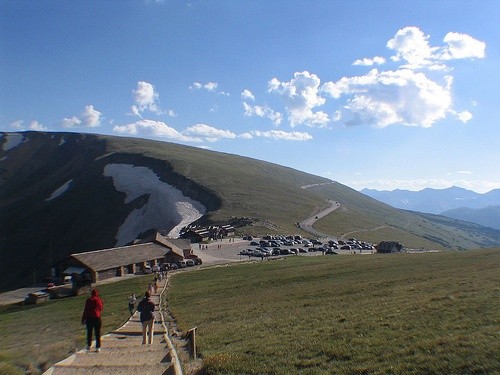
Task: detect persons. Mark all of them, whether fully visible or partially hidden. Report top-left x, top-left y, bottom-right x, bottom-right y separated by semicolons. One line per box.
217;244;219;249
229;239;231;242
266;252;269;258
147;270;169;297
128;293;136;316
82;289;103;353
233;239;234;242
261;254;264;261
220;245;221;249
206;245;207;249
202;245;204;249
137;291;156;345
199;244;201;249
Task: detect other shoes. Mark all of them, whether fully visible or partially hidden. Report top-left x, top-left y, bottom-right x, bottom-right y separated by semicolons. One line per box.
95;348;101;353
141;337;153;345
86;345;92;350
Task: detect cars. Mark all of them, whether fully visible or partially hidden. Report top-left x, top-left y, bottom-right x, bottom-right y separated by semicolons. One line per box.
240;235;373;255
